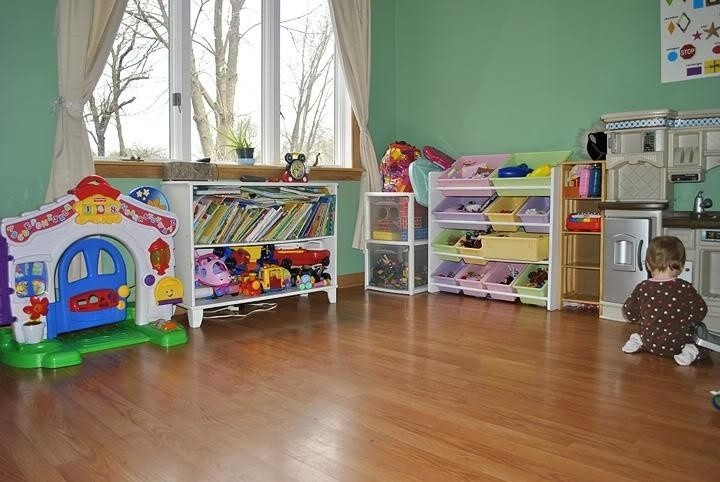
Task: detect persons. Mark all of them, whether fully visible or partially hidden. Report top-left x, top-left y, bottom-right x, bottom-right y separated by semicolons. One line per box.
621;235;714;367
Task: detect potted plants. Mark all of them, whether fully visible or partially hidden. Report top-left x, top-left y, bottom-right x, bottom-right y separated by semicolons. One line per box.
207;119;255;159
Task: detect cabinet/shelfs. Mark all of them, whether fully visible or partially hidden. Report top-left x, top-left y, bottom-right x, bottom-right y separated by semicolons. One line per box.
557;160;605;318
423;154;562;313
164;177;338;329
364;192;427;297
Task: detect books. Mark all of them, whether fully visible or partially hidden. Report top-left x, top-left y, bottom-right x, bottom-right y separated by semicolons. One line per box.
192;185;337;245
